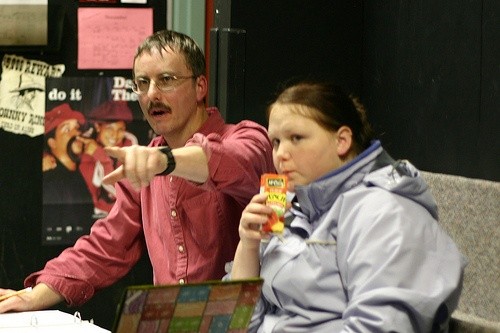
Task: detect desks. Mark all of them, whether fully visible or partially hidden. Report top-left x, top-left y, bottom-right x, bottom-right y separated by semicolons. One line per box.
0;308;110;333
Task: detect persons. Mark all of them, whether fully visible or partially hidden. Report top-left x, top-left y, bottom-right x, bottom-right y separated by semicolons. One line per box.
1;30;277;314
87;101;136;218
43;103;94;225
220;84;469;333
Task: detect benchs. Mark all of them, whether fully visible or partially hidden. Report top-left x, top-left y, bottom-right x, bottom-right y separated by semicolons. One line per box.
419;170;500;333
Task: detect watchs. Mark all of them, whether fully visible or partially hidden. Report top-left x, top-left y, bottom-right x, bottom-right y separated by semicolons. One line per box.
155;146;177;177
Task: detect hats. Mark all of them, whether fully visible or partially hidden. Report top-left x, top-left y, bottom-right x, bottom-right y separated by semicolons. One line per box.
86;101;134;121
46;104;86;133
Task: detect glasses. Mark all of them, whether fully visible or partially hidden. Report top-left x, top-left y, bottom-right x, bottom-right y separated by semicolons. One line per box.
130;74;196;94
